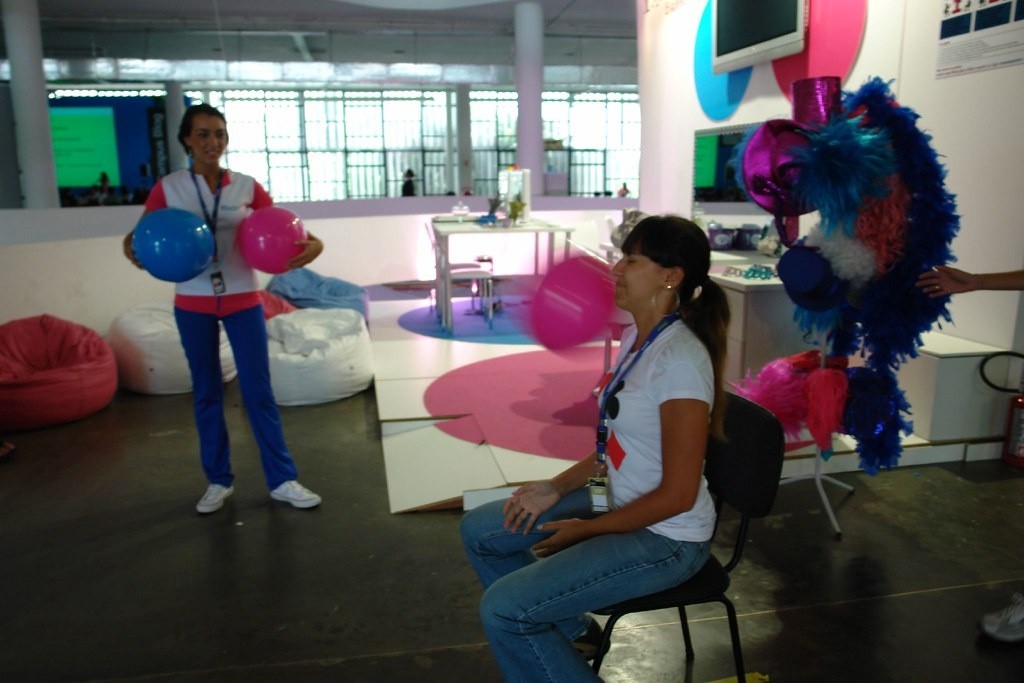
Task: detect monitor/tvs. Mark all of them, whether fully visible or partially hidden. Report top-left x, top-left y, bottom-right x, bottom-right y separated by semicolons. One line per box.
711;0;810;72
690;121;772;218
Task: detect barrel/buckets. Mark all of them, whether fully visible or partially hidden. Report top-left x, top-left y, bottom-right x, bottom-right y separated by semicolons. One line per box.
710;229;734;249
737;228;762;250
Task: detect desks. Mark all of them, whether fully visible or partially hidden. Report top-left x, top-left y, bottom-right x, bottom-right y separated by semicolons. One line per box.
698;250;808;389
431;216;572;329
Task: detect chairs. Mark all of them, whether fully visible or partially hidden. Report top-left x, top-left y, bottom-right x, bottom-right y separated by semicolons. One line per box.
591;392;785;682
422;226;494;332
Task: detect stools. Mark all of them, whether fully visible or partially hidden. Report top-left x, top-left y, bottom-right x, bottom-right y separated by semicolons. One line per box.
109;302;237;395
256;263;377;407
1;312;119;426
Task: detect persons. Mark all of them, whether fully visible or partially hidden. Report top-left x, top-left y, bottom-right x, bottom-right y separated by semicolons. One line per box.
916;266;1024;642
402;169;416;196
94;173;115;197
618;183;629;197
460;216;731;683
123;102;320;513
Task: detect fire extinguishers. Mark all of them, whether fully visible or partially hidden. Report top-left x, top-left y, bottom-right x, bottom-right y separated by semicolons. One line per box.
979;351;1024;469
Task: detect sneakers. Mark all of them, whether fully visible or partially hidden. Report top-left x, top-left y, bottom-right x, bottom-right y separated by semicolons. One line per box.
982;592;1024;640
196;483;234;512
571;617;610;660
270;480;321;507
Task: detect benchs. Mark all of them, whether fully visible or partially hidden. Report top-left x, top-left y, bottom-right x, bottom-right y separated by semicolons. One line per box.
850;326;1011;439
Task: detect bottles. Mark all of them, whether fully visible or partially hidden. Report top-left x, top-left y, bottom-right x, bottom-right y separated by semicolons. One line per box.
691;202;704;230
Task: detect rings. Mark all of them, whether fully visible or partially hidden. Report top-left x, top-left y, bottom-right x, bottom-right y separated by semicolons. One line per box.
936;285;940;290
